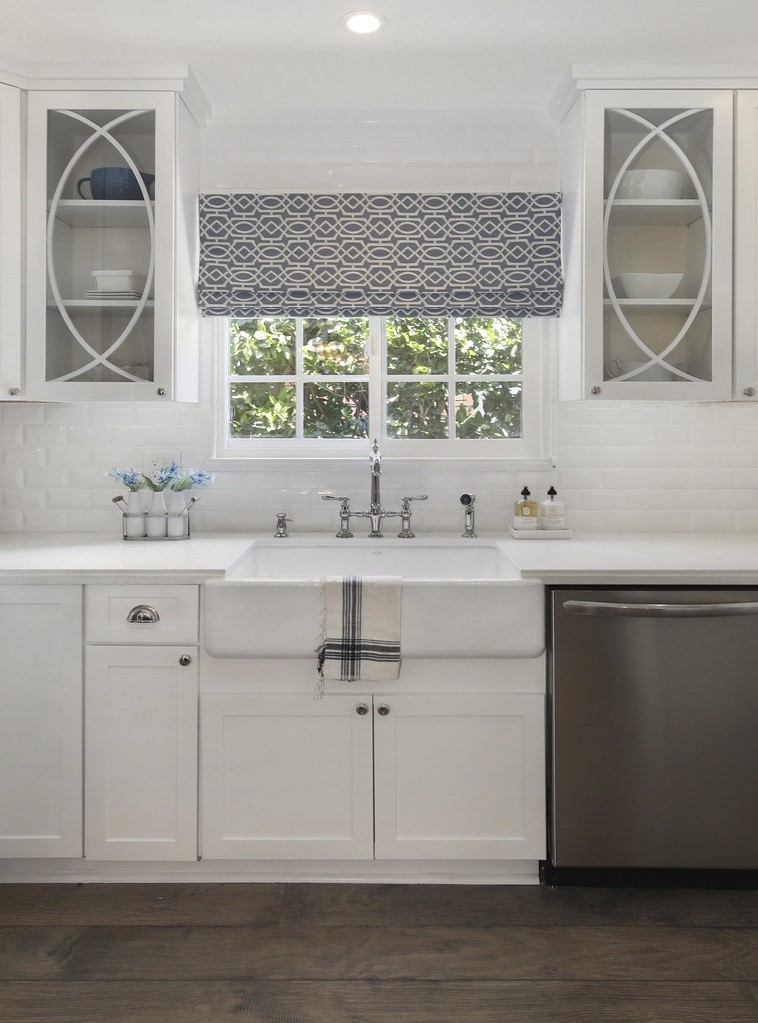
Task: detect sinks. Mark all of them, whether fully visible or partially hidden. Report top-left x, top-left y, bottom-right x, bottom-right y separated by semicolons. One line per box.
201;530;547;661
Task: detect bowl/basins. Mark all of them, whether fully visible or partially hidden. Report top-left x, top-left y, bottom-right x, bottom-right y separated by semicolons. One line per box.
604;273;683;299
607;168;683;199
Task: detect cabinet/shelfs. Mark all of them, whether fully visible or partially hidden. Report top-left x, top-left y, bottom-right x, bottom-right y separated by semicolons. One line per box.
0;582;200;884
547;63;758;407
198;693;547;884
0;55;213;405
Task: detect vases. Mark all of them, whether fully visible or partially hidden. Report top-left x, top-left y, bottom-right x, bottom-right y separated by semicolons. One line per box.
167;491;189;536
146;491;166;536
126;491;146;537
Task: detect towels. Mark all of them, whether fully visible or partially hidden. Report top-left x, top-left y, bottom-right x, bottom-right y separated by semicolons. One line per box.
313;573;405;701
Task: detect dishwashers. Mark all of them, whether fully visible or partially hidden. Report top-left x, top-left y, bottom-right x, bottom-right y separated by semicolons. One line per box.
550;587;758;886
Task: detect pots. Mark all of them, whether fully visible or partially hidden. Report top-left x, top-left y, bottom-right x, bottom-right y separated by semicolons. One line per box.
78;166;156;200
604;359;682;381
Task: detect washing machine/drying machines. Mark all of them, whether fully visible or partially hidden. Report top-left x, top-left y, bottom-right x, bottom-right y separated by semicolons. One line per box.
547;584;756;872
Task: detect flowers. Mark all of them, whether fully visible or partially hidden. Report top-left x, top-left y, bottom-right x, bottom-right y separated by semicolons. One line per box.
140;462;179;492
104;466;146;491
170;464;217;491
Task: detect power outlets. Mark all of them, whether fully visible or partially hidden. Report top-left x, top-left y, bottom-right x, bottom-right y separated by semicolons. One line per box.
157;449;181;467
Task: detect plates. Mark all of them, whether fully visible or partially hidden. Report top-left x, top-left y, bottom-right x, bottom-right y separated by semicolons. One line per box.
509;525;573;538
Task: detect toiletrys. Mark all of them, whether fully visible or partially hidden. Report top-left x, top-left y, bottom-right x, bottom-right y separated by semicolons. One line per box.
513;485;538;530
539;486;566;530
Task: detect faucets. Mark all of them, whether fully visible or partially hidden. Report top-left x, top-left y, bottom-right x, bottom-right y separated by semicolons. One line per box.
274;513;292;538
368;437;384;538
459;493;477;538
